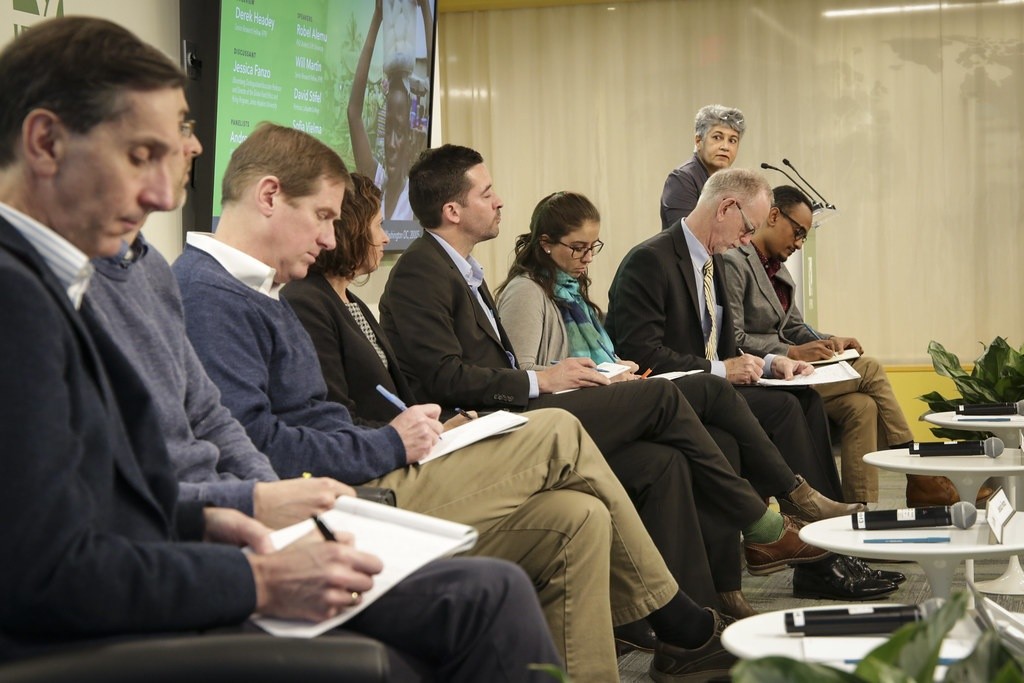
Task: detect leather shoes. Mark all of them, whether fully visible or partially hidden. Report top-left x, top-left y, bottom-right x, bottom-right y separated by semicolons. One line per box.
720;591;757;619
744;513;833;577
613;617;658;653
778;475;868;524
792;554;906;599
906;474;992;509
649;606;740;683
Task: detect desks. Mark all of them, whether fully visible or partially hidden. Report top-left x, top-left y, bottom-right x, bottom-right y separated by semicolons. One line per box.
862;447;1024;610
720;604;1024;682
924;407;1024;449
798;510;1024;614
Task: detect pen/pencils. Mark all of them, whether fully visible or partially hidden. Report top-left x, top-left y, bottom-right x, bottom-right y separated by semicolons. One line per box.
738;348;744;355
311;514;337;541
303;472;312;478
376;384;442;440
804;323;836;357
641;363;656;379
550;361;610;373
455;408;473;420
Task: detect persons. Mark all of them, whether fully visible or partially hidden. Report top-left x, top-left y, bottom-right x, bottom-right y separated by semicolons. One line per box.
79;71;359;530
378;144;836;613
660;104;746;231
279;172;738;683
492;190;865;620
346;0;433;221
173;122;741;681
604;168;907;601
713;184;993;508
0;15;565;683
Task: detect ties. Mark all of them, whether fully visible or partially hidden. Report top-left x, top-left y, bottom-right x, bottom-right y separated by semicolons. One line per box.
704;259;718;360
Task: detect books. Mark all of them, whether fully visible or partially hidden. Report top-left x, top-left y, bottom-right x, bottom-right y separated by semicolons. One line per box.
806;349;860;365
234;493;479;639
756;361;861;386
418;410;529;465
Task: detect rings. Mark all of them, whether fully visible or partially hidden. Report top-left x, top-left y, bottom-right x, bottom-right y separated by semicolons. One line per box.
351;592;357;605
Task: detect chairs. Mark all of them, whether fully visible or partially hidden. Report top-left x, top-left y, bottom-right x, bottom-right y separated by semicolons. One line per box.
1;476;434;683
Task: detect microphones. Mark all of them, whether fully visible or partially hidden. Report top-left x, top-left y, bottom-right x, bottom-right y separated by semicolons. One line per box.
851;501;977;530
956;399;1024;415
909;437;1004;457
783;160;830;206
785;597;951;636
761;163;818;205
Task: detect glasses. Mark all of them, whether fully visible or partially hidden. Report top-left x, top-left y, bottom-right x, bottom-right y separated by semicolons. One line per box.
779;210;808;243
179;119;197;142
723;198;756;237
549;233;604;259
